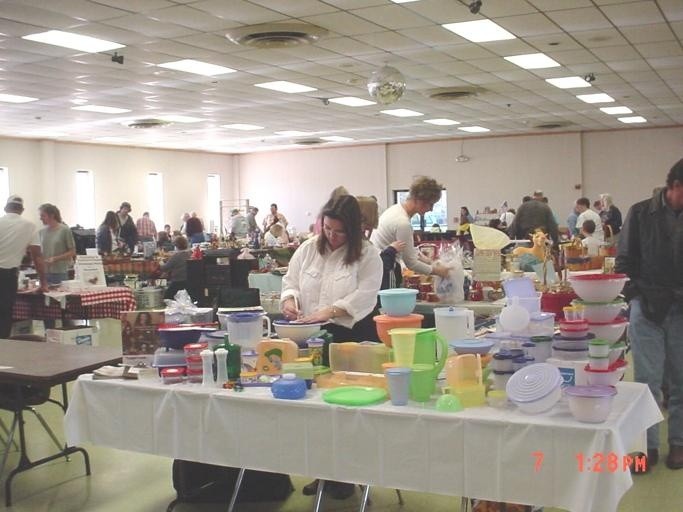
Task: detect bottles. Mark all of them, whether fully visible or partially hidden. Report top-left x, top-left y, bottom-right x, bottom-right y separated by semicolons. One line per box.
199;349;215;387
215;348;230;384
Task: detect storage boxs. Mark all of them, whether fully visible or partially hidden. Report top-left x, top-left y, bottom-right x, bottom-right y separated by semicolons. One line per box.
46;325;99;345
121;308;167;356
11;320;45;340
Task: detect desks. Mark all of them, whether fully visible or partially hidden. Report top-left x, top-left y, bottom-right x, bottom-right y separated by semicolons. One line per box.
102;255;170;281
248;270;285;293
13;286;137;334
259;290;509;323
62;368;664;511
0;338;123;507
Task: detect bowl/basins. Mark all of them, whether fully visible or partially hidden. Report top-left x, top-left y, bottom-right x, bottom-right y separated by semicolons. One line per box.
590;339;610;357
550;347;590;361
505;364;564;414
563;302;587;321
590;355;610;370
558;326;589;339
378;287;420;317
449;338;495;393
551;273;630;383
563;382;618;423
552;333;594;351
584;364;621;388
559;318;589;328
373;314;424;348
272;317;325;344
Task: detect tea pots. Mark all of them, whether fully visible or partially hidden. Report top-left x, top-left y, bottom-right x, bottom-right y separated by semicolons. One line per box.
389;326;453;384
227;313;273;349
434;305;476;345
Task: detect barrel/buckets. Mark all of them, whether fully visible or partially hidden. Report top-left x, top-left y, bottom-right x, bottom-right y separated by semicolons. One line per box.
410;328;448;395
225;313;271;354
433;306;475;351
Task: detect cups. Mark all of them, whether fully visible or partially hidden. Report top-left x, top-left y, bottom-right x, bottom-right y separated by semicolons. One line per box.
410;364;435;402
386;367;409;406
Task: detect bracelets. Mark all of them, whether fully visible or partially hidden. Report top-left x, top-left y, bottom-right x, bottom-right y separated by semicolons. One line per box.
333;308;336;322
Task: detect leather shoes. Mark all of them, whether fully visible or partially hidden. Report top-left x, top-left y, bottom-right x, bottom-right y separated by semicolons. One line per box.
329;481;356;499
303;479;329;496
632;448;660;475
667;443;683;471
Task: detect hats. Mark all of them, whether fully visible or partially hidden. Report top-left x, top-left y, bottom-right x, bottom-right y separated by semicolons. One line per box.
5;194;25;206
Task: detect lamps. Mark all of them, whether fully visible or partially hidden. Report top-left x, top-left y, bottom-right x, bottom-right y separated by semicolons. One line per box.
533;122;569;128
225;22;328;49
112;52;123;63
292;138;325;145
128;118;169;128
585;73;594;82
469;0;481;14
424;87;478;101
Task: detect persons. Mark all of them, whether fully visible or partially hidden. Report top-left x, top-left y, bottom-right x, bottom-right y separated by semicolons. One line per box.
313;187;348;235
369;174;455;287
307;224;314;240
2;195;288;338
355;195;407;343
461;191;621;260
281;194;384;499
615;157;683;475
